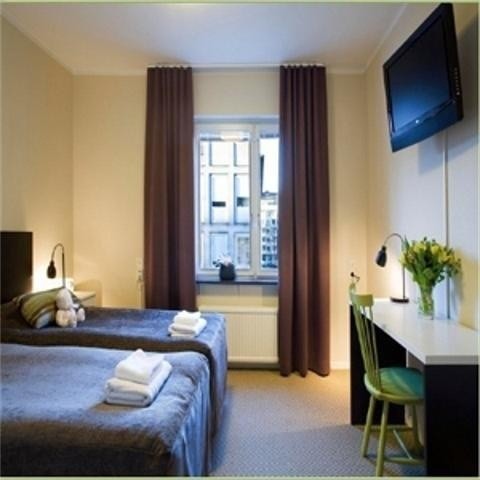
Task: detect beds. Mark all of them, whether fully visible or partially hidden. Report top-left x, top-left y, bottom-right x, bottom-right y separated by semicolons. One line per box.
0;344;215;476
0;231;229;416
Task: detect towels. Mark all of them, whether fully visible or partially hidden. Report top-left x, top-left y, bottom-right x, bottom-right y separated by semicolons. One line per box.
174;308;201;326
114;348;165;385
168;318;208;340
104;361;172;406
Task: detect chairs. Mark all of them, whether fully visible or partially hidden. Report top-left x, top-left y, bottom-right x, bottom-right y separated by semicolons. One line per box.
350;282;427;476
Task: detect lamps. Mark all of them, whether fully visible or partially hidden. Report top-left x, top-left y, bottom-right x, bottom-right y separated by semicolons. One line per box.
47;243;67;288
375;234;409;303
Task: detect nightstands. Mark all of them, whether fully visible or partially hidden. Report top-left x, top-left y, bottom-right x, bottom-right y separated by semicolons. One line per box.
74;290;96;307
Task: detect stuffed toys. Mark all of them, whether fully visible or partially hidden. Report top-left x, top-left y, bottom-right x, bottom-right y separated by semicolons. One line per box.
56;289;85;329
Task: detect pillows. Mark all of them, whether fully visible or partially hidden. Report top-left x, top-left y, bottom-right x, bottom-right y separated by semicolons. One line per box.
13;287;84;330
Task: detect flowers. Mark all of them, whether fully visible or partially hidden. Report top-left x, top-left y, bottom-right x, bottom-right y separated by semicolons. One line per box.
211;250;233;266
399;238;465;312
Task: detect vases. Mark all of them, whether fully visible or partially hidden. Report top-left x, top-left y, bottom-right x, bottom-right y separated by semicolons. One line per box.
219;265;236;280
416;292;435;320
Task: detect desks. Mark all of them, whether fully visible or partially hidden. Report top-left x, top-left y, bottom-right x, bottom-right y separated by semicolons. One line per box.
348;297;478;476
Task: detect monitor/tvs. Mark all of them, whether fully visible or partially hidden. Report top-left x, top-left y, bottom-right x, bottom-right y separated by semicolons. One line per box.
383;3;464;152
